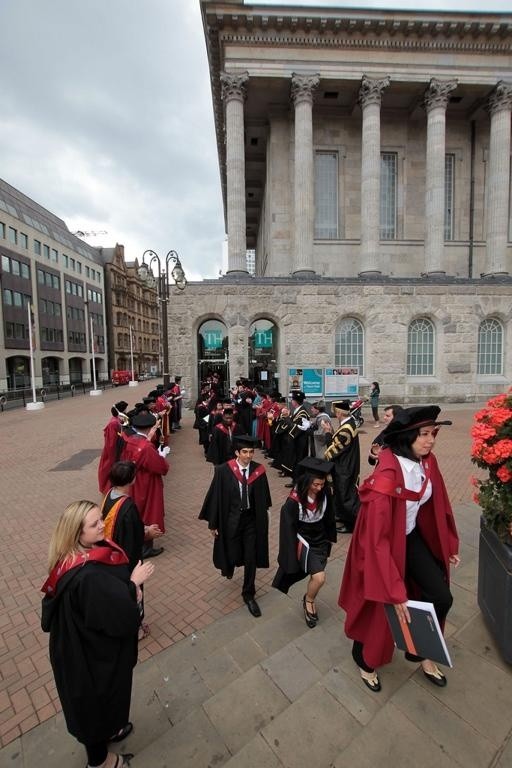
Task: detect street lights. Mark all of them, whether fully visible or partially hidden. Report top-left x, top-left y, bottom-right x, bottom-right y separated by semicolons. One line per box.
139;247;187;393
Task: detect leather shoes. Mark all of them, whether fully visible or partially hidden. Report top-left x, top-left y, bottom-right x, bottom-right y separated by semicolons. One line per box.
336;519;350;533
303;594;318;628
113;723;132;742
424;663;447;686
113;754;133;768
244;599;260;617
146;547;163;558
360;668;381;691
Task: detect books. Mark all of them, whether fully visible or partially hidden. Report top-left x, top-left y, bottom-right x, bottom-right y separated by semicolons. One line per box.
384;600;453;668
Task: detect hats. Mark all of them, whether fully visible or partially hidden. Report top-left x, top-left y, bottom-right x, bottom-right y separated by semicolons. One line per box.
201;381;286;413
235;434;261;449
313;397;327;409
331;399;352;413
290;390;306;400
383;406;452;442
297;456;334;478
112;376;181;430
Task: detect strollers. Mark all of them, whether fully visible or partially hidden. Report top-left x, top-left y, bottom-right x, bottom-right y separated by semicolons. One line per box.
334;398;368;428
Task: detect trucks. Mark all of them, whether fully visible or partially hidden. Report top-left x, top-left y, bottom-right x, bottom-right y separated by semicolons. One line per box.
111;370;138;386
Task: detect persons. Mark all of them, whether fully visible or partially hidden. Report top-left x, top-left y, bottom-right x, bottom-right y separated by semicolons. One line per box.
369;382;380;428
321;399;360;533
272;455;338;629
193;375;242;465
198;434;272;617
367;404;404;465
100;376;184;558
42;500;158;768
229;377;331;487
98;461;163;642
338;405;460;692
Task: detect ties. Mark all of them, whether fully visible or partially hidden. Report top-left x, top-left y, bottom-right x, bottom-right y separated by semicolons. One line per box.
242;469;248;509
